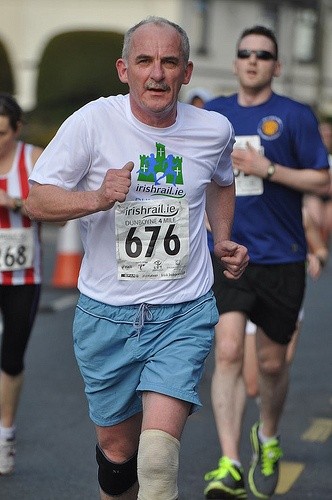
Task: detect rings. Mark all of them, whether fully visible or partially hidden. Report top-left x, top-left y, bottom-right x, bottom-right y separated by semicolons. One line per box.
240;267;243;272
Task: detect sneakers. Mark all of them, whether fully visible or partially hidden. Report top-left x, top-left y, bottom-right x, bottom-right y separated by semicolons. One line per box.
246;421;283;500
203;456;248;500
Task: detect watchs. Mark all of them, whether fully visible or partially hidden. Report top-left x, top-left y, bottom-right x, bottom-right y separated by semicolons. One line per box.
265;161;276;184
12;197;21;214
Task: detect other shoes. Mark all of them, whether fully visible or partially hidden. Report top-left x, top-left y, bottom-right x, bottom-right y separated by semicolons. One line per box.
0;441;18;474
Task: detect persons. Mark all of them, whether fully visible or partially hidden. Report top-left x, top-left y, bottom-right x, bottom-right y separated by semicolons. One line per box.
185;86;332;414
23;17;249;500
183;26;330;500
0;96;44;476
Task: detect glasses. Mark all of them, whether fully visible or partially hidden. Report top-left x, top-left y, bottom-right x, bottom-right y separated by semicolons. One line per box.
238;49;276;61
